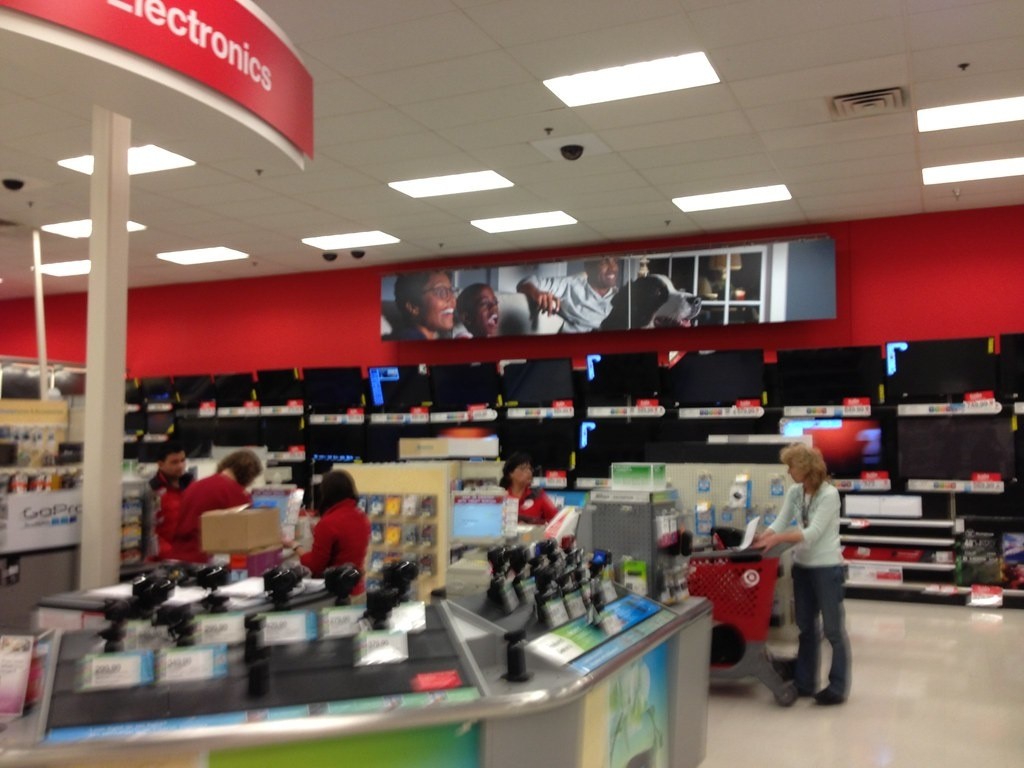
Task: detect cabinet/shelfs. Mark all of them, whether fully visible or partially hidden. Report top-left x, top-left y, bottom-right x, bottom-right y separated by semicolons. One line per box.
840;518;1024;609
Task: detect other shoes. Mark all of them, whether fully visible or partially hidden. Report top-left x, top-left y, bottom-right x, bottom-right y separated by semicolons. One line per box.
815;690;843;705
792;683;818;697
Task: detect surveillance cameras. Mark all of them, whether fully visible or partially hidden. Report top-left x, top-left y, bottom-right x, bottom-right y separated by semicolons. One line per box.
351;250;365;258
3;180;24;191
560;145;583;160
323;252;337;261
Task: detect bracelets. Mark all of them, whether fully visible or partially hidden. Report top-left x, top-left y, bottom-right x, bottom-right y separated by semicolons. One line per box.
292;542;302;551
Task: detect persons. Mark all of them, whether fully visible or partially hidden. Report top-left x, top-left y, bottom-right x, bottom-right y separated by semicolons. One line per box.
452;283;500;340
748;443;852;706
517;258;620;334
155;450;262;564
498;452;559;526
148;441;196;563
380;270;461;341
281;469;366;607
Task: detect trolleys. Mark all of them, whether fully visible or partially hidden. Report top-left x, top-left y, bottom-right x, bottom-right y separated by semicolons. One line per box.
686;537;799;708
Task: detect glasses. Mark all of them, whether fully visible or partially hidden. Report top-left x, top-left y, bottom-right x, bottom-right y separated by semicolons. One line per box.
422;286;459;300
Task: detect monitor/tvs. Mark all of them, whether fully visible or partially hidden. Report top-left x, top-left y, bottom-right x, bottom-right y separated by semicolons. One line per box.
118;331;1024;539
0;358;88;401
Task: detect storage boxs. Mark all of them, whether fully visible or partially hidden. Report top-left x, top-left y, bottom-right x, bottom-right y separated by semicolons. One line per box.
590;488;679;504
204;545;283;581
0;490;82;554
611;462;667;491
200;502;281;553
